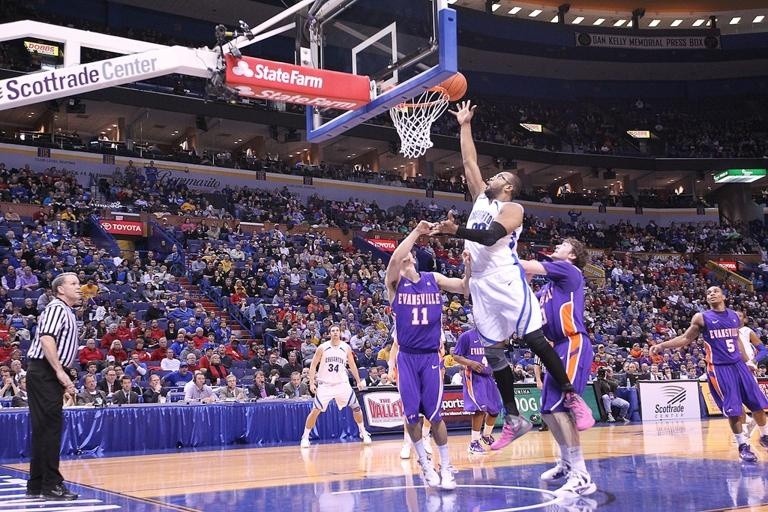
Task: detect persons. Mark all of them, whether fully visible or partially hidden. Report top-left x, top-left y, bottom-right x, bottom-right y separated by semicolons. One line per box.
2;158;768;424
433;86;767;161
385;324;432;457
25;270;83;500
400;456;506;512
429;98;596;450
384;219;472;488
517;236;599;498
451;321;499;455
732;305;766;439
649;284;768;461
724;456;768;509
301;444;372;512
538;478;599;512
299;323;373;447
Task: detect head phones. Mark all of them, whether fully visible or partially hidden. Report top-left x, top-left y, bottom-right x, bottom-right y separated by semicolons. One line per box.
225;375;238;386
193;373;206;383
253;372;268;382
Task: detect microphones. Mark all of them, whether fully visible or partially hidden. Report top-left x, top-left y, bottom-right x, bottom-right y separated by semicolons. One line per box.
203;382;206;384
262;380;268;385
227;385;232;389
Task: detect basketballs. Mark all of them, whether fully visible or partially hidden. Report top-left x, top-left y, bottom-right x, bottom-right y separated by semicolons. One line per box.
441;73;466;101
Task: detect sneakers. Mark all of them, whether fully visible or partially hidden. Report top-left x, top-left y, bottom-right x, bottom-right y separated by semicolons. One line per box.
540;464;572;481
553;468;598;497
482;433;494;445
359;432;371;444
468;436;488;456
417;457;441;487
301;435;310;448
490;416;533;451
738;436;768;462
438;463;458;491
607;412;630;423
399;441;412;460
25;485;80;501
564;392;595;432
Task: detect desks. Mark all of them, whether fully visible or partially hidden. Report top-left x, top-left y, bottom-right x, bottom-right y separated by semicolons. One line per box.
637;379;768;421
0;398;358;460
355;380;607;436
